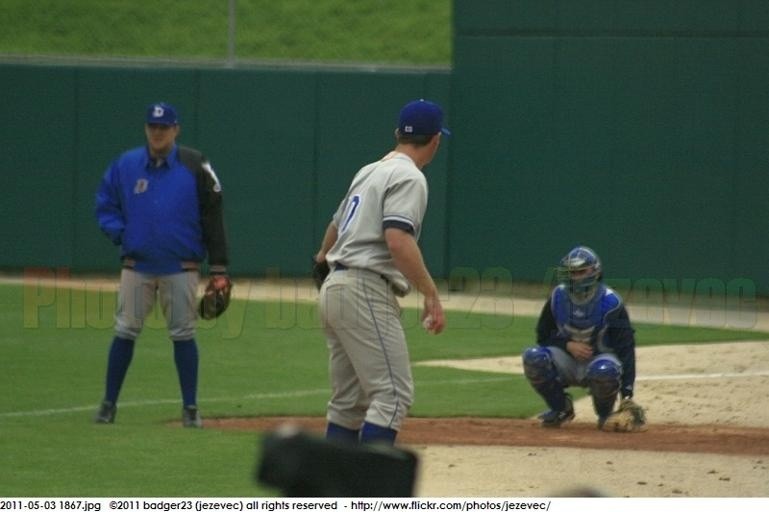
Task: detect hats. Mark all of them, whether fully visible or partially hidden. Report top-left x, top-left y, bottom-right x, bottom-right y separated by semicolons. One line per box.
400;98;451;137
146;102;176;128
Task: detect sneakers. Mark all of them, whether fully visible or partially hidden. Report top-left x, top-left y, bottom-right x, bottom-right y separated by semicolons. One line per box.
183;405;200;428
94;402;116;423
539;406;574;425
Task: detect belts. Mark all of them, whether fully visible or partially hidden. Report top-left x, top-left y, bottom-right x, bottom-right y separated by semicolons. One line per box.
334;264;390;285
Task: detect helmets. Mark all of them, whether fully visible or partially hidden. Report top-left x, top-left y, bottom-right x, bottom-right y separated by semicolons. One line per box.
556;245;600;298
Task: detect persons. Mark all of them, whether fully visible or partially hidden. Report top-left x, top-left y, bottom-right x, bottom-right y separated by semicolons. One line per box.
93;99;232;429
522;246;646;432
311;98;451;448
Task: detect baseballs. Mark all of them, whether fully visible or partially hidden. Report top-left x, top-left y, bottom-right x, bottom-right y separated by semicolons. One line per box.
423;315;434;331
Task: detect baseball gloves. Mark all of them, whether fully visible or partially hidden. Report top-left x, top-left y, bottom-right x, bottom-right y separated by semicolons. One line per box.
602;400;646;431
312;257;330;292
199;275;232;319
